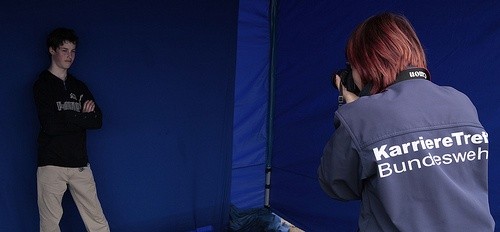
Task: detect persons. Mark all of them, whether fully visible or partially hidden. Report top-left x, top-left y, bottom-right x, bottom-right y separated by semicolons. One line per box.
33;28;111;232
317;13;496;232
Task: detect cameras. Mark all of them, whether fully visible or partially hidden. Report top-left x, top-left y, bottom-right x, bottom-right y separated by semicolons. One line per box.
330;64;362;97
